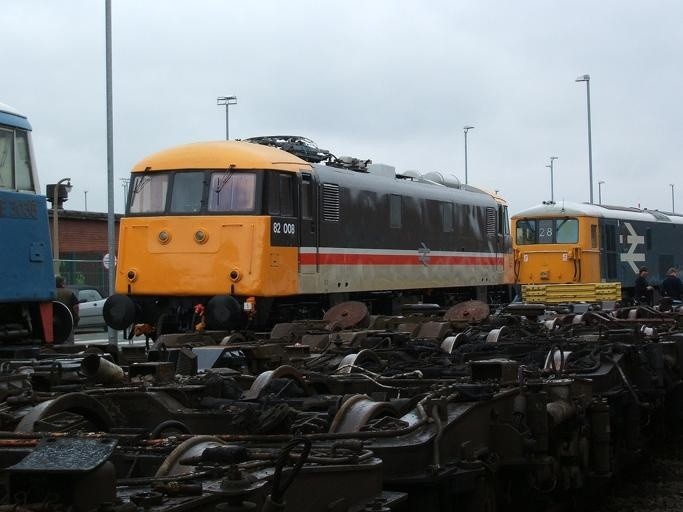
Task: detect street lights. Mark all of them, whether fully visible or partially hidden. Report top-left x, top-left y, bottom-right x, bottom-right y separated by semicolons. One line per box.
574;72;594;205
669;184;674;214
598;181;605;205
546;156;559;201
463;125;475;184
217;95;237;142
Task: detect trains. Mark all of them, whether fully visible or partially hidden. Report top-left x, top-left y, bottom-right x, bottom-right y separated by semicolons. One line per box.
114;133;518;330
510;199;683;290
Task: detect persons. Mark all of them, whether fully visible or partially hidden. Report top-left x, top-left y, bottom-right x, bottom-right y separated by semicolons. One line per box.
53;274;79;345
634;266;654;305
659;266;683;298
